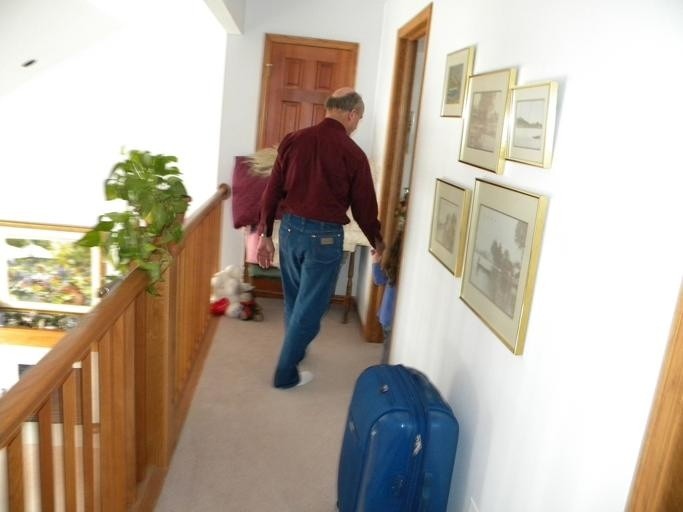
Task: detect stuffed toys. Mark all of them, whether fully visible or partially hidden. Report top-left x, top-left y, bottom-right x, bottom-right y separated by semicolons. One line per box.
210;265;255;319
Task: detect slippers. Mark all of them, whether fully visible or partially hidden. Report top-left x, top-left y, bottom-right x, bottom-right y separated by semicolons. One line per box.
295;371;314;388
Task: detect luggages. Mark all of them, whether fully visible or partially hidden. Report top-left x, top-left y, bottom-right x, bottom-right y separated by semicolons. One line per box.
336;362;461;512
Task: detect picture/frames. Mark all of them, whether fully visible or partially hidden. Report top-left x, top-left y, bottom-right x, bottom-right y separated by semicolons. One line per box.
440;45;474;117
0;220;107;318
459;176;551;358
457;66;518;172
429;176;475;277
503;81;558;171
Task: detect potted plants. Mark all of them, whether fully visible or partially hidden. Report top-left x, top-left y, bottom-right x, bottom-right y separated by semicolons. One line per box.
76;146;192;297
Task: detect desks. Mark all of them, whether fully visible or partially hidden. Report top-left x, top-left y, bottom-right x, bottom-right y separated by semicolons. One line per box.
241;212;365;324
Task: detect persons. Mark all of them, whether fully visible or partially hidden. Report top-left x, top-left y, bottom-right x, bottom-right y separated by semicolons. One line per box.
369;223;402;365
256;87;386;389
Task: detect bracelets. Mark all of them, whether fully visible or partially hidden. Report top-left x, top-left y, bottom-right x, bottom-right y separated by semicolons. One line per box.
260;233;271;238
